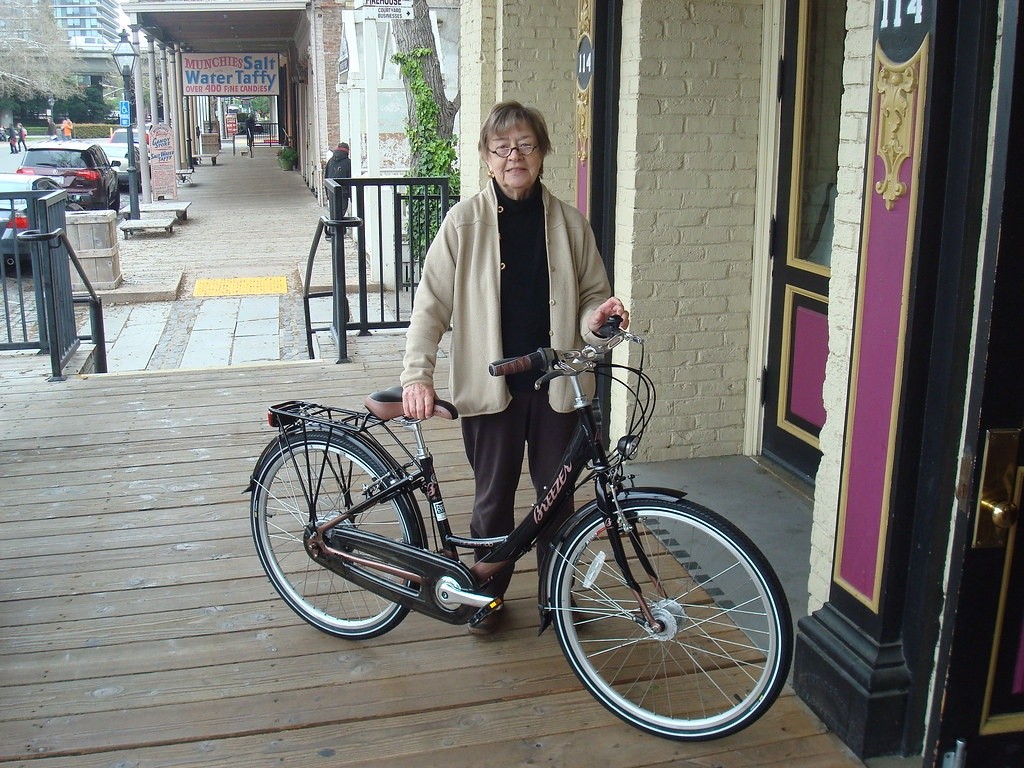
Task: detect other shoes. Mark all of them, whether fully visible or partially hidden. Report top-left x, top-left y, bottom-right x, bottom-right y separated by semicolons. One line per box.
549;595;582;635
468;592;504;634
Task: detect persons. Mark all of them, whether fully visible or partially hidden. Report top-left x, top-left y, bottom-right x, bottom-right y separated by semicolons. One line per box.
0;125;6;142
15;123;27;152
247;114;254;141
401;103;630;635
325;142;351;239
8;124;18;154
62;119;74;139
47;117;57;135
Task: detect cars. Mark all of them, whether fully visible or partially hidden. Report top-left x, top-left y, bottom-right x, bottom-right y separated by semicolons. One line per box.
100;143;141;192
16;140;121;214
109;129;150;167
0;173;85;266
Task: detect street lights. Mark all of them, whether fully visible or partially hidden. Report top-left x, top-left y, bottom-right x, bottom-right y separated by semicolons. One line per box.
111;29;140;220
47;95;55;121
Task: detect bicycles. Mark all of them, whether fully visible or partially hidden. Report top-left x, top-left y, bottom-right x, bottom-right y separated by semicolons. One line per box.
240;314;795;744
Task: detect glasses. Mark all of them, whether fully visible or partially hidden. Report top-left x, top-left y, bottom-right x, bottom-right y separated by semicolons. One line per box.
487;143;539;157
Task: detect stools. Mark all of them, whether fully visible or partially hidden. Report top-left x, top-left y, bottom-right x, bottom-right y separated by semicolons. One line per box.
120;218;175;240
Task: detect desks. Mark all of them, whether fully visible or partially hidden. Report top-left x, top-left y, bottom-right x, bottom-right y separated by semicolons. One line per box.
119;202;192;225
192;154;219;166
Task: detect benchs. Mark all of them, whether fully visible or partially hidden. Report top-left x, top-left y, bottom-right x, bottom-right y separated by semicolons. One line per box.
176;169;193;187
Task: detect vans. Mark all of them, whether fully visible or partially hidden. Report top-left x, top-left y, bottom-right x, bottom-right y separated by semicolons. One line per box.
68;36;117;53
